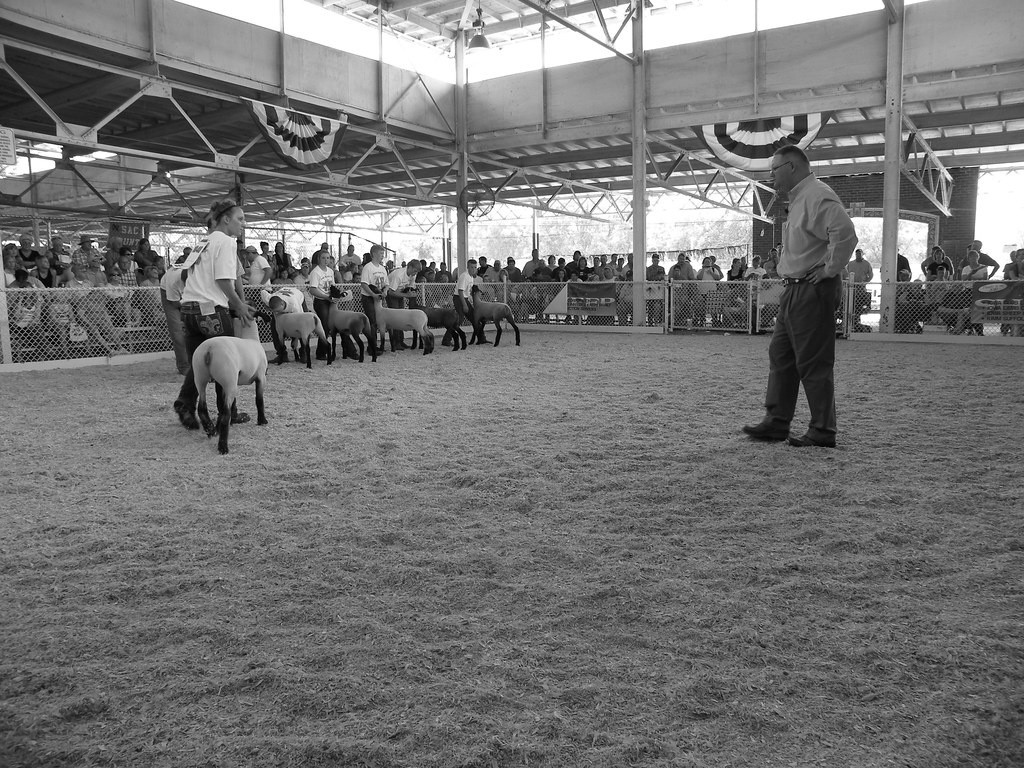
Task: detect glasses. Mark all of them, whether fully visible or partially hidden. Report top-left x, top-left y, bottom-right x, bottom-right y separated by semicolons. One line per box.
770;160;790;173
124;253;134;256
112;273;121;277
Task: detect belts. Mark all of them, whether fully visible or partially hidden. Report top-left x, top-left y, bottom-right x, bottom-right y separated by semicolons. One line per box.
782;276;800;286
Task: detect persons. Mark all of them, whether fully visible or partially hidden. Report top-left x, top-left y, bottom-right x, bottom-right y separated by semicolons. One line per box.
2;234;1024;356
309;249;360;360
385;259;423;350
174;200;256;430
160;263;192;376
207;217;246;303
741;144;858;448
360;244;390;356
261;286;308;364
441;259;493;347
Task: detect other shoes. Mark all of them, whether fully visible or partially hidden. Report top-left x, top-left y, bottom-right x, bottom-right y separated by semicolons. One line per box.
299;347;309;364
442;340;453;346
269;353;289;363
743;423;789;440
479;338;493;345
788;435;837;448
393;340;411;350
348;353;360;360
317;354;328;361
367;347;383;356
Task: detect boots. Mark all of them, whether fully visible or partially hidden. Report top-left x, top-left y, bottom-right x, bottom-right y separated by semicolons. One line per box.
215;388;250;424
174;370;201;429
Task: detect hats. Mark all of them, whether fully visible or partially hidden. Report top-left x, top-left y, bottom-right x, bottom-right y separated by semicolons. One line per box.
77;235;95;245
301;263;309;268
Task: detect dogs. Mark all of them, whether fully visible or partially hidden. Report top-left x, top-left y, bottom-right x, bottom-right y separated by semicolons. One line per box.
369;284;434;355
401;287;467;351
271;310;331;372
468;285;520;347
192;300;271;455
328;284;377;362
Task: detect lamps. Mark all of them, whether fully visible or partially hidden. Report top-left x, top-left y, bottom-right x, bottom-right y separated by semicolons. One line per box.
467;0;491;53
